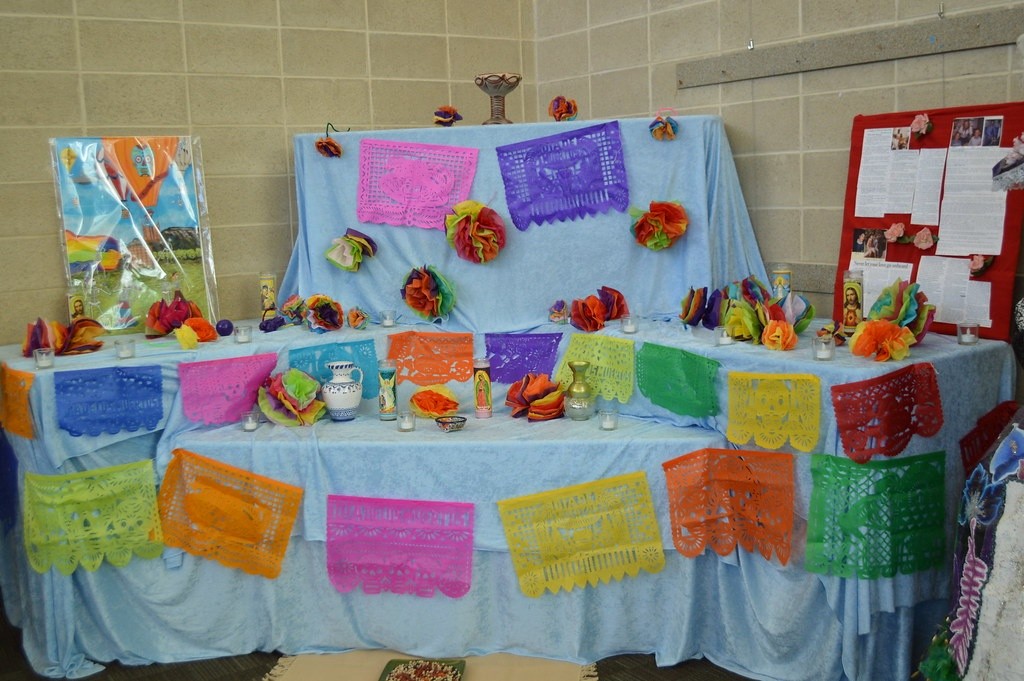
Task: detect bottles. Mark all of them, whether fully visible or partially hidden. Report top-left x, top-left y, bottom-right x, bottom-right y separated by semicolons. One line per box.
377;360;397;420
771;263;791;310
473;358;492;419
260;272;276;322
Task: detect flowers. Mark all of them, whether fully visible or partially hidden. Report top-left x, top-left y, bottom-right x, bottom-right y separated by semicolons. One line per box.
24;94;996;427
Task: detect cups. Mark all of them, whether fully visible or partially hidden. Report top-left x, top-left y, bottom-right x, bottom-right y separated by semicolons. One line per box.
622;315;637;332
33;349;54;368
114;339;136;358
958;324;979;345
812;338;835;361
714;326;733;345
397;412;416;431
381;311;396;326
242;412;259;430
599;409;617;430
234;326;252;343
